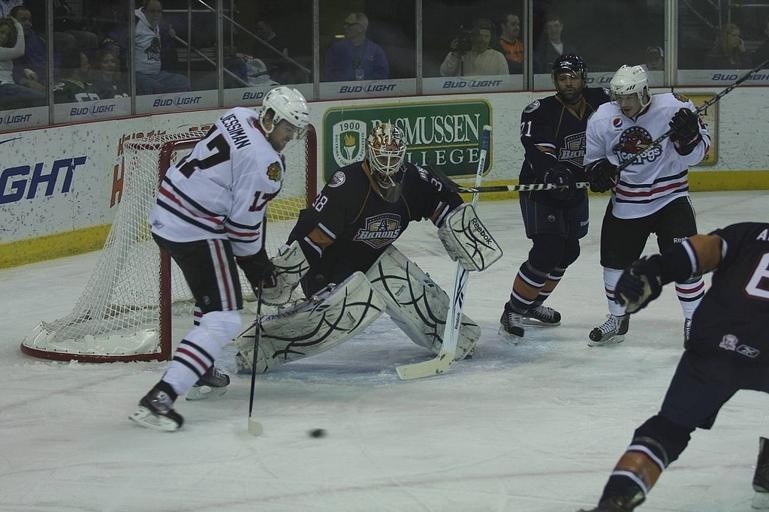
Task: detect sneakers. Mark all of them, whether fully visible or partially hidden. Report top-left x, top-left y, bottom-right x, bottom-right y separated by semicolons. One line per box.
195;366;230;387
589;311;629;341
500;301;561;337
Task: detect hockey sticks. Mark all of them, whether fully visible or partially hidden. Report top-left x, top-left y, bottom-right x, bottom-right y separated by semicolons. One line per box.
426;158;590;194
397;126;490;379
247;210;266;437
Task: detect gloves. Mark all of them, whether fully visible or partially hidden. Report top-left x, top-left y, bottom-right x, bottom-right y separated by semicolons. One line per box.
584;158;621;194
541;167;576;201
234;249;275;294
669;108;702;154
614;242;692;315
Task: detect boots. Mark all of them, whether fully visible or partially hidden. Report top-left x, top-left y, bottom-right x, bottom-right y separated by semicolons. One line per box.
138;380;182;425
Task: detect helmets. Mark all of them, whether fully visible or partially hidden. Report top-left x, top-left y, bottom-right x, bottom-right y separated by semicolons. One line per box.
552;56;587;99
367;123;410;176
259;86;310;138
610;65;653;109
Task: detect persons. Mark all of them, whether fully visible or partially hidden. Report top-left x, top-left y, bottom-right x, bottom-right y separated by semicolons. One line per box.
582;63;711;349
580;221;768;512
236;121;503;373
499;53;610;338
127;85;309;432
